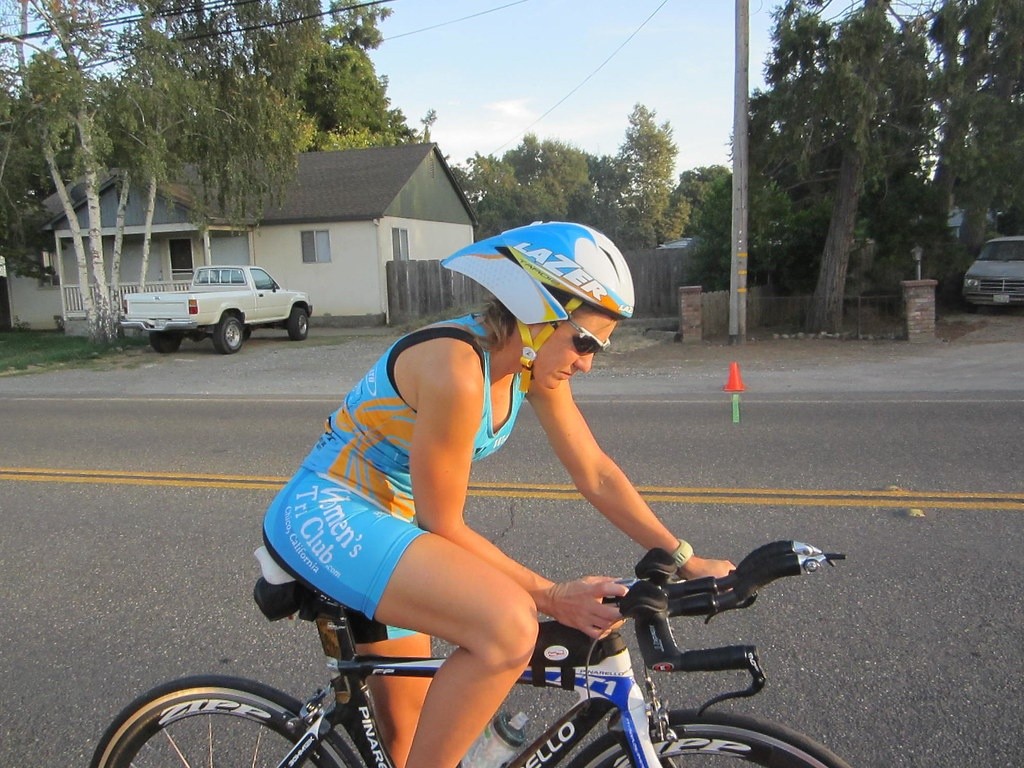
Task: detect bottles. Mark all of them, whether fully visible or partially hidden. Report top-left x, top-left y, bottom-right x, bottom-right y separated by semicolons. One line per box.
456;712;530;768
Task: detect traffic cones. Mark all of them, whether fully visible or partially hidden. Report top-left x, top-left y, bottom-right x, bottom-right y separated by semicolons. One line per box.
722;362;745;391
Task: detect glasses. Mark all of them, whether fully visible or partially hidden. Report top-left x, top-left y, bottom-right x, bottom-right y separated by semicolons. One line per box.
565;319;613;354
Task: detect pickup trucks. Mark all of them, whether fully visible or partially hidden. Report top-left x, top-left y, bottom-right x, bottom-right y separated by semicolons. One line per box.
118;265;311;355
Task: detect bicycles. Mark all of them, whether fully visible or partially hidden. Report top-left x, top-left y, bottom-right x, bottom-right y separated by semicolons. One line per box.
87;545;850;768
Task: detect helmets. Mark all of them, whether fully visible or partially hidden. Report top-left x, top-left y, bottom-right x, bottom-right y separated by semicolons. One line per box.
440;221;635;326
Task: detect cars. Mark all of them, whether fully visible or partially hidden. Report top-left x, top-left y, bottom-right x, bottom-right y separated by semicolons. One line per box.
961;236;1024;307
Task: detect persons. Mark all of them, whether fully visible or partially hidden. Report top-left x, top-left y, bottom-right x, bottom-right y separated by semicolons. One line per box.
262;221;737;768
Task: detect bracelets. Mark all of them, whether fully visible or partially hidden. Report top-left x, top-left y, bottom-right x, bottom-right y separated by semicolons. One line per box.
670;538;694;568
